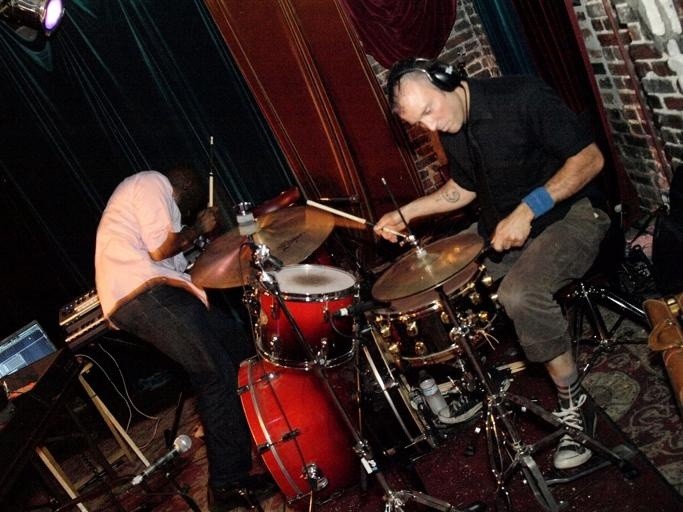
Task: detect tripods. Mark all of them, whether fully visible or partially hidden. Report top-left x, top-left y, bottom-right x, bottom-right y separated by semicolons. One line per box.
435;283;632;512
237;199;459;511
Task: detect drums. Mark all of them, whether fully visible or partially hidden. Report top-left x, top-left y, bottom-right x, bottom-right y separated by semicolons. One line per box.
254;263;359;370
372;261;497;367
353;319;427;449
238;356;364;509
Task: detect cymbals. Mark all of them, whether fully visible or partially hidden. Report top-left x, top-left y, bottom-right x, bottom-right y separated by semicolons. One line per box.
192;207;335;289
372;233;484;300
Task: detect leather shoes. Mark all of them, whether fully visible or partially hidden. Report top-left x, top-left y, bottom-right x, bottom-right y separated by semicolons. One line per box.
209;474;280;512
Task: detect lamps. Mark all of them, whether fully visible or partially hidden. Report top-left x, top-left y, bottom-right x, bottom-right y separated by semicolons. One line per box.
1;0;65;36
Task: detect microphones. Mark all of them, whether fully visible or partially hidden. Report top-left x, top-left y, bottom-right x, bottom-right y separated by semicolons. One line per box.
254;242;284;270
130;433;194;486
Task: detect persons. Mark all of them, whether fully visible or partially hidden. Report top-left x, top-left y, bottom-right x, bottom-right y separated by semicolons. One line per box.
94;147;280;512
374;65;623;469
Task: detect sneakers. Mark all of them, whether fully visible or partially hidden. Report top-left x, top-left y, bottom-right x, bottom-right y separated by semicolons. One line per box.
551;387;599;470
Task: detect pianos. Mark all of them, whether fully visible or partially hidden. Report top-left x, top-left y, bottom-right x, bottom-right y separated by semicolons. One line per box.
64;252;200;348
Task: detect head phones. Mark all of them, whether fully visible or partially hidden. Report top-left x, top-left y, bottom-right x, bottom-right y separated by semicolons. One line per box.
388;60;463;92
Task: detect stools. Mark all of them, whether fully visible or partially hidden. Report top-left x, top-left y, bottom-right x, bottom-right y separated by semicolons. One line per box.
556;254;653;393
